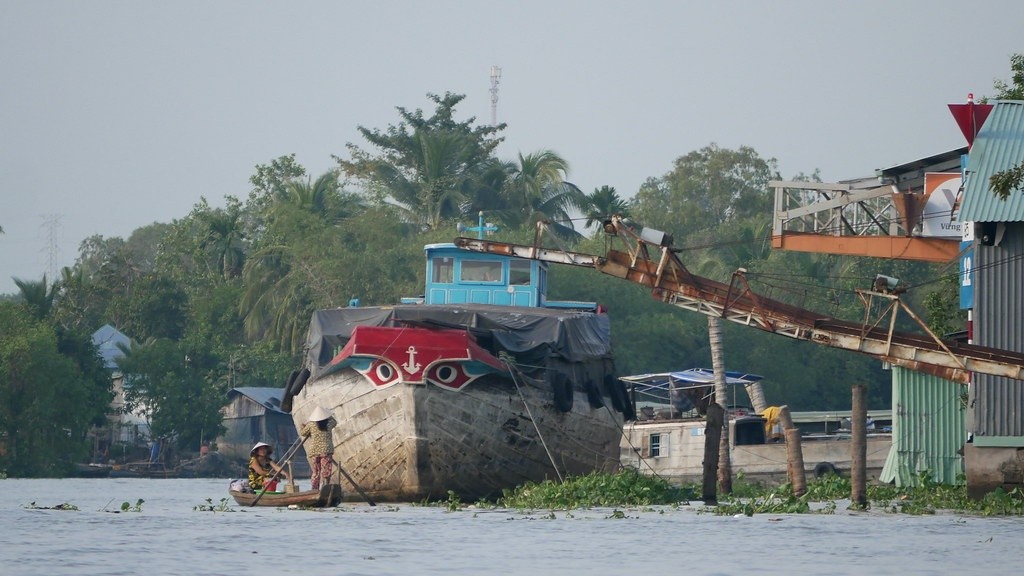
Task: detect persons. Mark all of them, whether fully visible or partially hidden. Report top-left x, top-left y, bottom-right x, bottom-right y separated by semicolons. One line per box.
248;442;294;490
299;406;337;490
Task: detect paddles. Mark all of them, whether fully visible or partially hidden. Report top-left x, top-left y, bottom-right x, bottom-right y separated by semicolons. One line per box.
250;433;310;508
330;458;376;508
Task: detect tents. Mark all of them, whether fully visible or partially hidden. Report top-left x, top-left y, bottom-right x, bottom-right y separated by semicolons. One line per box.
621;369;765;422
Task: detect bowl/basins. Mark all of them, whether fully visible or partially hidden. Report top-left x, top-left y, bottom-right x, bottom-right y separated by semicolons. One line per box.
254;490;286;495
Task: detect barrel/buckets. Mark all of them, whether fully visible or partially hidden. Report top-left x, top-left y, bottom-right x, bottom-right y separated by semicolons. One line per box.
264;479;276;491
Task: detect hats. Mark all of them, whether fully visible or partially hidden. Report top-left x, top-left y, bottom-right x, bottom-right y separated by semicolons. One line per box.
309;406;332;421
250;442;273;457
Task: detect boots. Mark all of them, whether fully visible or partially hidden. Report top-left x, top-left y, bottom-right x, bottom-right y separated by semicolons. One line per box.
322;477;329;487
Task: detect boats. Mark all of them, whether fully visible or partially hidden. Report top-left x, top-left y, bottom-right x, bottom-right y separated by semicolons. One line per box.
292;206;625;508
231;479;321;509
74;435;251;480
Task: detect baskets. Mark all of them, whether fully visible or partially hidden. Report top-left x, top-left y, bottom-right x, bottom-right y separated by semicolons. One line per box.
284;485;299;493
